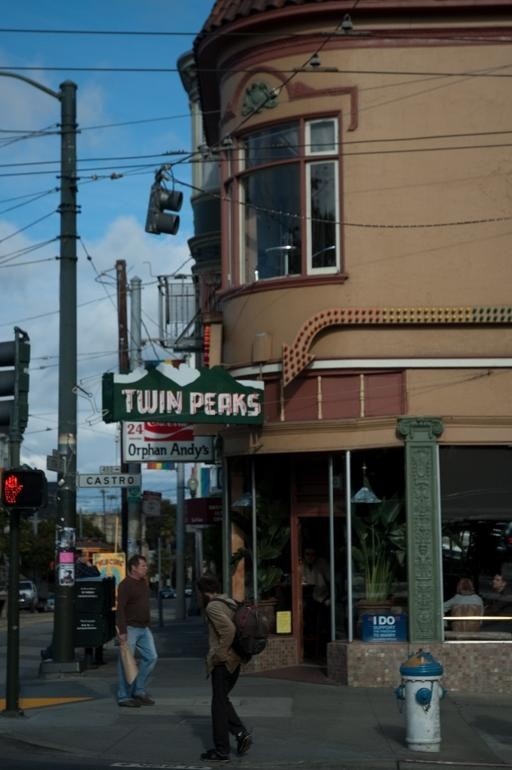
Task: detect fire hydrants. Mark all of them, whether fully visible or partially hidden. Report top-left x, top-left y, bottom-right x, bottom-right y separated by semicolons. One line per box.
394;649;448;753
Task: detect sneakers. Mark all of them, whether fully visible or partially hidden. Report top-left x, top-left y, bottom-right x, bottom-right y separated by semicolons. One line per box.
120;699;143;708
200;747;231;763
235;730;254;757
133;694;156;706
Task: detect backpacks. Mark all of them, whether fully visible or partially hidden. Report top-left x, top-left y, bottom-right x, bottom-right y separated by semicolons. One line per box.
202;597;271;661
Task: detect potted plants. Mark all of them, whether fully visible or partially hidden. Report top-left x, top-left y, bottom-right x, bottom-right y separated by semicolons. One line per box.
353;527;397;631
228;490;292;634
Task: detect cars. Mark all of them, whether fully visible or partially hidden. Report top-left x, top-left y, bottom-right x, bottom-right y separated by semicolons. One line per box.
19;580;55;613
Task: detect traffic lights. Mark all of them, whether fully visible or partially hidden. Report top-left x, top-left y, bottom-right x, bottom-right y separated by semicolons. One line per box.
145;182;182;235
0;325;48;515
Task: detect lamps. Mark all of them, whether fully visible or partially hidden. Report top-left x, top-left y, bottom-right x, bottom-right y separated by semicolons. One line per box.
349;452;380;505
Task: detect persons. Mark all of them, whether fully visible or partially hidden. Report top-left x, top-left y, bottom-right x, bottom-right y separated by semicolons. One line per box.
443;570;512;640
301;544;330;589
196;571;254;763
114;554;159;708
